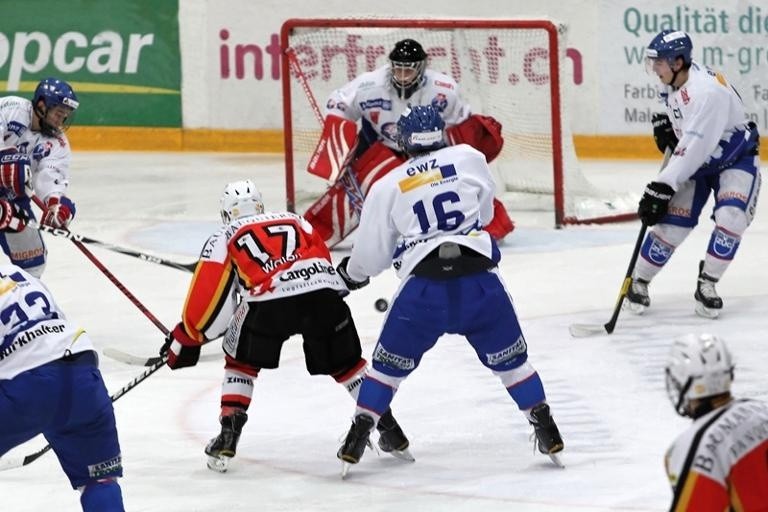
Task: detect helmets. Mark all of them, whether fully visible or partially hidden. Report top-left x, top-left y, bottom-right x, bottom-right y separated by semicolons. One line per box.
661;331;736;417
645;29;692;67
218;178;265;227
397;104;447;155
388;40;429;100
30;76;80;139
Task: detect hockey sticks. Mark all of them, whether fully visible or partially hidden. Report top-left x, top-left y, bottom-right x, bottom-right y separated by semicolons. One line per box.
569;143;672;339
102;327;229;365
0;355;170;469
25;221;198;273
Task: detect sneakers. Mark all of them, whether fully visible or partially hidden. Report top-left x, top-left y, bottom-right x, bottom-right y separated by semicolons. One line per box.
692;260;722;310
626;273;649;306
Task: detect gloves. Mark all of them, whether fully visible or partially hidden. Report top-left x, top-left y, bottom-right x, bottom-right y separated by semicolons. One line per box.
637;182;675;225
0;154;32;198
158;324;201;371
334;255;369;290
40;191;76;231
650;113;678;154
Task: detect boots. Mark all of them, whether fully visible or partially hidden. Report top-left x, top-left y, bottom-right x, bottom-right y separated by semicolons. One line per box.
204;411;247;457
528;404;564;456
335;413;373;464
376;410;410;454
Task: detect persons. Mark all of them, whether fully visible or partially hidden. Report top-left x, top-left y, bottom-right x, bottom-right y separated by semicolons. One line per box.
0;76;80;280
627;29;760;308
334;105;564;466
661;333;767;512
0;254;126;512
162;176;417;463
302;38;516;252
